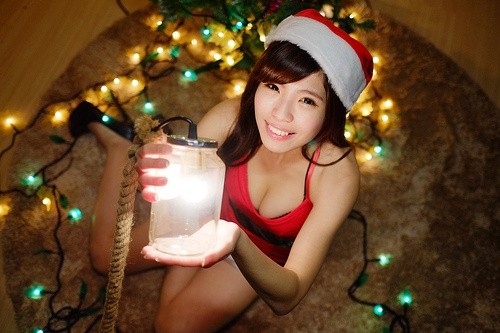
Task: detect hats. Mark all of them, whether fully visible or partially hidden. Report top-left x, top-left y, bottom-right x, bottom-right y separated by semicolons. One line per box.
264;8;374;114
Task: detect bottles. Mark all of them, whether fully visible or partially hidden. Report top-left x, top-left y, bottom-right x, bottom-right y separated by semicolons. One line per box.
149;135;226;256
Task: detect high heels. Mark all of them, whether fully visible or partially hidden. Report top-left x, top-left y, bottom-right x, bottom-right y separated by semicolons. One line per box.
68;90;135;143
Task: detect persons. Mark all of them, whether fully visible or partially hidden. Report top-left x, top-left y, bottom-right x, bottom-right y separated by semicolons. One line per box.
68;7;374;333
